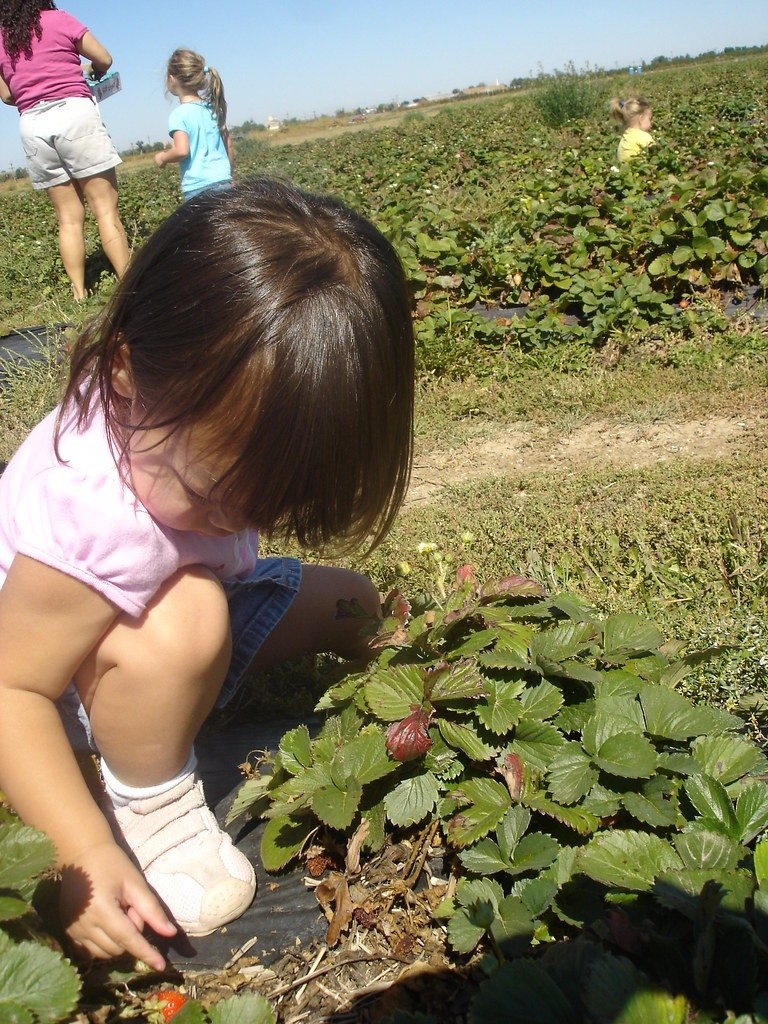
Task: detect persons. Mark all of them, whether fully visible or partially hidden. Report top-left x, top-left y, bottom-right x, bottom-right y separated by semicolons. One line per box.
0;0;133;301
0;176;418;973
611;95;654;161
154;49;235;203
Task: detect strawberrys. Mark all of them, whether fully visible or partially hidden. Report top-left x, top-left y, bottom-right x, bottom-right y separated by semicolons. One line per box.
142;990;189;1024
514;274;521;286
680;299;689;308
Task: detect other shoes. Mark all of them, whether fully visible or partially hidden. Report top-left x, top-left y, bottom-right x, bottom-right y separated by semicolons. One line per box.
86;753;256;935
248;662;330;724
